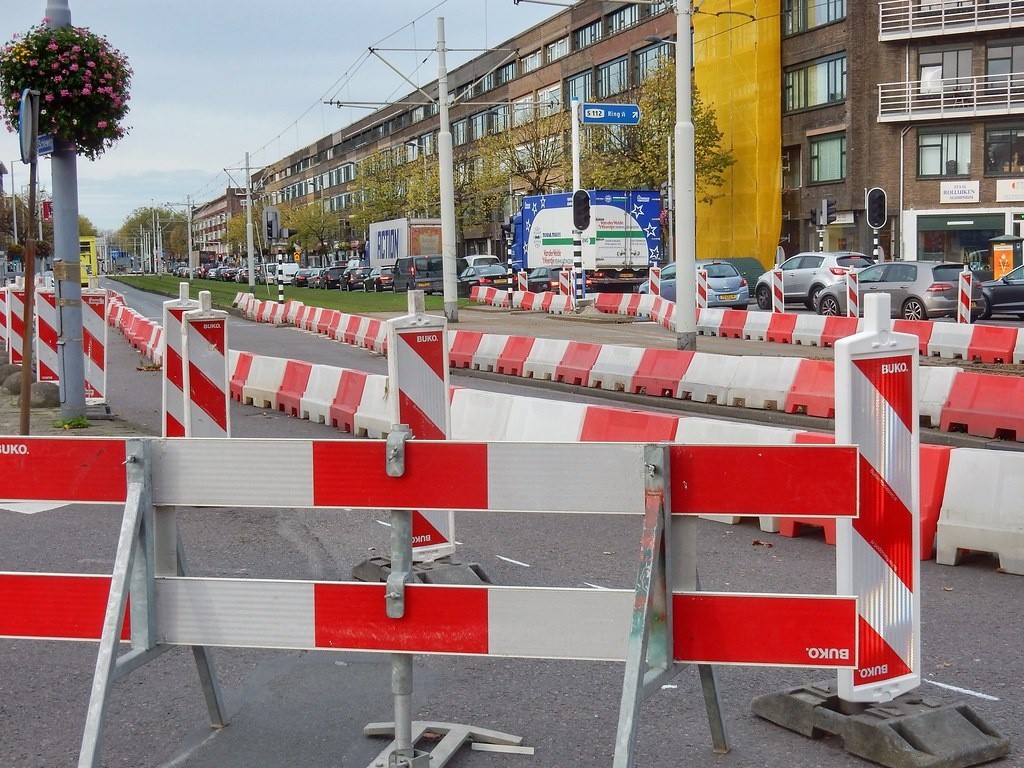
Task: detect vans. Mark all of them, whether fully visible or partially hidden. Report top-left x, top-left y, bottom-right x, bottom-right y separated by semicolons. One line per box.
257;264;279;285
392;255;443;294
463;254;500;267
273;263;299;286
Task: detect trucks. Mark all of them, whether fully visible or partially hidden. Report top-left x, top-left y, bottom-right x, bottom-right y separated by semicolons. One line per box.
191;250;217;268
500;188;661;293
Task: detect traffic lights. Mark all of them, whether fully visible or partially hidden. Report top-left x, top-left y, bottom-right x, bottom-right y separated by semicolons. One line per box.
572;189;591;230
811;208;821;226
866;187;888;230
821;199;836;225
501;216;516;246
338;219;351;241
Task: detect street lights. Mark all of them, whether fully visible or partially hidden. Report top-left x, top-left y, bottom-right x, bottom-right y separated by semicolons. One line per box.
36;153;52;241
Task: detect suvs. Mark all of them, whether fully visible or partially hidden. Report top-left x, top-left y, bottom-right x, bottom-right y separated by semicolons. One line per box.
977;264;1024;320
755;251;877;310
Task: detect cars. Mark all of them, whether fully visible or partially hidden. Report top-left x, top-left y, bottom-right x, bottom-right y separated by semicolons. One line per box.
307;269;325;289
291;269;314;287
460;264;518;294
638;261;750;310
320;266;347;290
167;266;259;284
816;260;985;324
526;266;591;295
339;267;372;292
362;264;395;292
